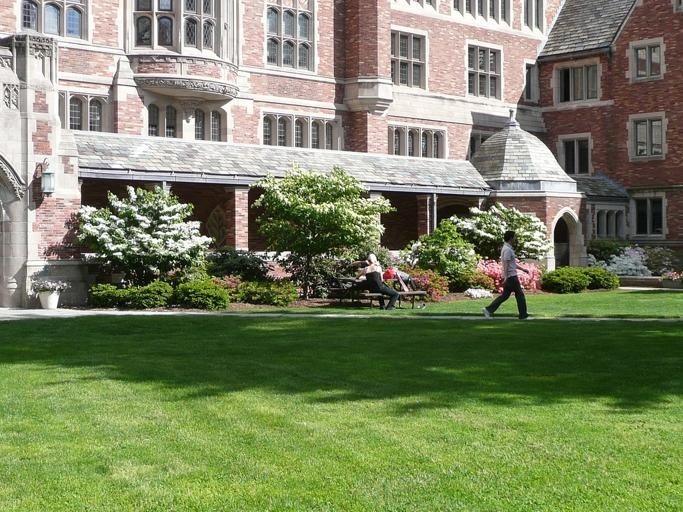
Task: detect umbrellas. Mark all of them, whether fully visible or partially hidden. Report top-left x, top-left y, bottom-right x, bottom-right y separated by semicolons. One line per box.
380;305;395;310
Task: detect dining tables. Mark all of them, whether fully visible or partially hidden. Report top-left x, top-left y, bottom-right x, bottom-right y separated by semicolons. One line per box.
31;281;72;308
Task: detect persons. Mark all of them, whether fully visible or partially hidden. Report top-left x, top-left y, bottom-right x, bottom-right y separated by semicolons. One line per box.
382;262;428;310
481;230;536;321
354;253;399;310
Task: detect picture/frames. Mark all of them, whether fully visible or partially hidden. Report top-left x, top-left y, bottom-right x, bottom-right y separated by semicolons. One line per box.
338;277;418;310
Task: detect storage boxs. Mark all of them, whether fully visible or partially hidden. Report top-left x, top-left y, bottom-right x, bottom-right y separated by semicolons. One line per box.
328;287;427;308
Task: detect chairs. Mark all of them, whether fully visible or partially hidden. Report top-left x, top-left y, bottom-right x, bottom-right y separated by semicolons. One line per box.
518;317;535;320
482;308;491;320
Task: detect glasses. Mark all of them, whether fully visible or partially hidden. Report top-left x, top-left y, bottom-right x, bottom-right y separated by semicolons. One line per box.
33;157;54;202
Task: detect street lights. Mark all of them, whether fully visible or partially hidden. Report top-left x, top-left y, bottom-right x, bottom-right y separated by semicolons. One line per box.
383;269;395;279
